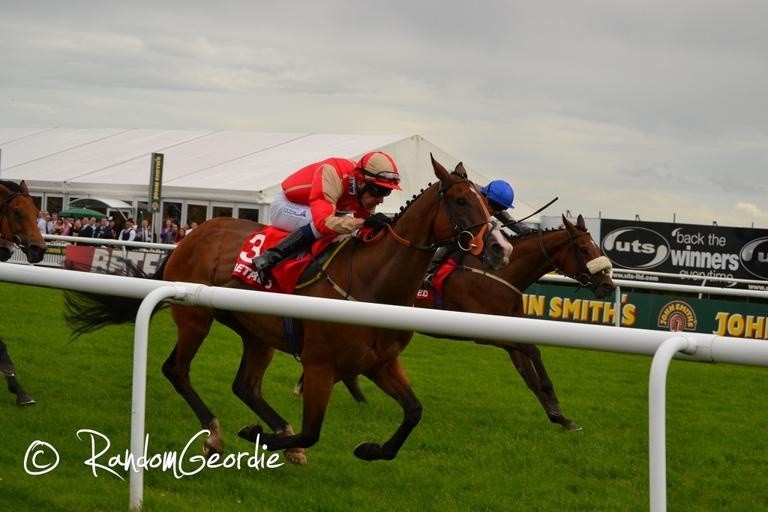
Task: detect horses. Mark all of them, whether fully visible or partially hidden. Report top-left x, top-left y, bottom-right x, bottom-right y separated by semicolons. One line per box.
0;178;48;406
61;151;514;465
292;212;618;433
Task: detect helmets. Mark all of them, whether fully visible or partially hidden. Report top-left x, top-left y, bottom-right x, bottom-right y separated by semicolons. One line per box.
478;178;517;210
351;151;404;192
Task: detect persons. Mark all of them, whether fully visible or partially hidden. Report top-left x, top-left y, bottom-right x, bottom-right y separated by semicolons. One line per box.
34;208;200;256
428;178;531;300
249;149;403;288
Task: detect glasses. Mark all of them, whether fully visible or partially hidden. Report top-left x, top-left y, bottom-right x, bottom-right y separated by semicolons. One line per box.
490;202;508;212
366;184;392;198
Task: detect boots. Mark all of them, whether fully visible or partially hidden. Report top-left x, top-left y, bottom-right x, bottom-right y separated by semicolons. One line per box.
421;245;452;292
252;227;313;286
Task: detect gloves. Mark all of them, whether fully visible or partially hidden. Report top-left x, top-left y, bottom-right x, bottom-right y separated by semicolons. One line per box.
362;211;392;229
